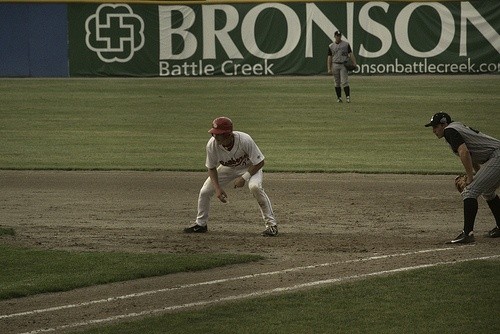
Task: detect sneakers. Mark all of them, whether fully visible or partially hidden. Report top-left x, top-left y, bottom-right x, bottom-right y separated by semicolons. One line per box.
445;230;475;244
490;227;500;237
261;225;278;236
183;223;207;233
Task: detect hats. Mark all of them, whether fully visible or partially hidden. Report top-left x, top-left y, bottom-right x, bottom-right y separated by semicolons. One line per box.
334;31;341;36
425;112;451;127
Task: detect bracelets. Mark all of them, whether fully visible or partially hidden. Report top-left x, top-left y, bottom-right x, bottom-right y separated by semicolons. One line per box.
242;171;251;181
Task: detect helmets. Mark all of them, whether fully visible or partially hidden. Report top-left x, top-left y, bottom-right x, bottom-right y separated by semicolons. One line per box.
208;117;233;134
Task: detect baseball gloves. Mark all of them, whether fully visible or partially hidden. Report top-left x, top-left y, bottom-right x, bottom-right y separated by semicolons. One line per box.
455;175;467;193
343;60;357;72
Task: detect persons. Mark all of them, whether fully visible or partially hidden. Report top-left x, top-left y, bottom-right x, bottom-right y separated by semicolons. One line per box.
184;117;278;236
328;30;357;103
425;112;500;244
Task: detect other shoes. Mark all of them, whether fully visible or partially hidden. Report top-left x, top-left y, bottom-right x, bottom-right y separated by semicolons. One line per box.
337;97;342;103
345;96;350;103
